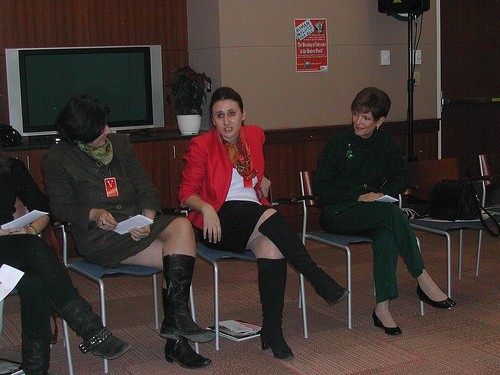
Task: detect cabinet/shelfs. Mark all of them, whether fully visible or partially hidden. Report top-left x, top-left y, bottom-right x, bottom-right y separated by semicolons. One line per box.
12;120;438;260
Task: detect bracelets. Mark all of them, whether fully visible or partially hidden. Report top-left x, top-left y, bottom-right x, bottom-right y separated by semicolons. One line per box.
26;225;41;237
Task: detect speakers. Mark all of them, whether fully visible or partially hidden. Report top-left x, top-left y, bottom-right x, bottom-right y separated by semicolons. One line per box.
378;0;430;16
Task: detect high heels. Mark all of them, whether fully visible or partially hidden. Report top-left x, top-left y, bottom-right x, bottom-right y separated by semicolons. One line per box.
416;284;456;308
371;307;402;335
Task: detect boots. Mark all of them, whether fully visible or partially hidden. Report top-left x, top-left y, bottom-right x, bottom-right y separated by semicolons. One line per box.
22;338;50;375
156;253;215;368
58;294;130;359
258;210;349;307
257;256;294;360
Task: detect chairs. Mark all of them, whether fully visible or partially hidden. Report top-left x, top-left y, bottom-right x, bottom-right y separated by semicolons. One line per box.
0;155;500;375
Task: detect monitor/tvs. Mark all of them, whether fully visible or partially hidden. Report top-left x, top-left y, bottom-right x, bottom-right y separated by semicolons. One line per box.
4;44;165;140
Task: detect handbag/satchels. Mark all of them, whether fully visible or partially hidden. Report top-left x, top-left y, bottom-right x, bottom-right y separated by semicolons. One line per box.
434;175;481;222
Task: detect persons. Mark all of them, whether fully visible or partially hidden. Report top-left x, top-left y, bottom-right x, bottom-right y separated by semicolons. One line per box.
177;86;348;360
314;86;457;335
0;158;132;375
42;94;215;368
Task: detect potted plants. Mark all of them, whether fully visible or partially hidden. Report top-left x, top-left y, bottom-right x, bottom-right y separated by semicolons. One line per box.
163;66;210;136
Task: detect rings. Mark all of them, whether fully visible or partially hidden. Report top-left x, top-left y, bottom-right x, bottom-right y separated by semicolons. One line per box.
101;221;106;226
